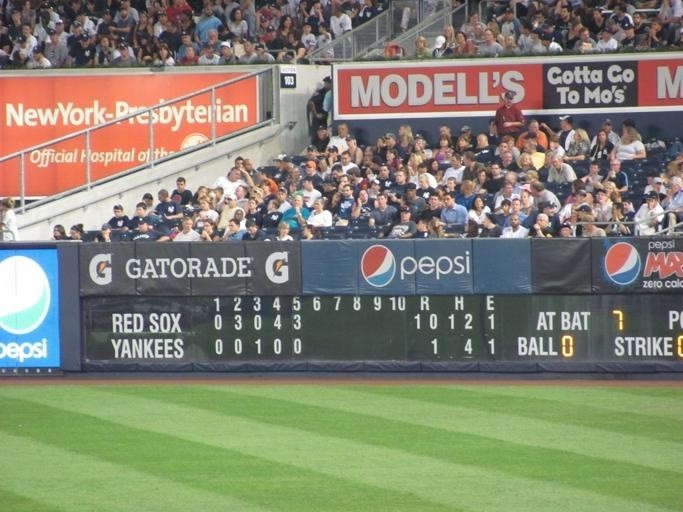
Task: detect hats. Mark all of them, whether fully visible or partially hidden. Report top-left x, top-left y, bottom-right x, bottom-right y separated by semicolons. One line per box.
435;36;445;48
558;115;571;122
574;203;590;211
486;212;498;224
622;23;633;29
504;92;513;100
220;41;230;47
642;190;657;199
79;33;88;40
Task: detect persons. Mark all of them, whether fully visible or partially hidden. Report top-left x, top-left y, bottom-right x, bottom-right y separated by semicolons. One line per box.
50;76;683;242
1;2;683;69
1;198;17;241
